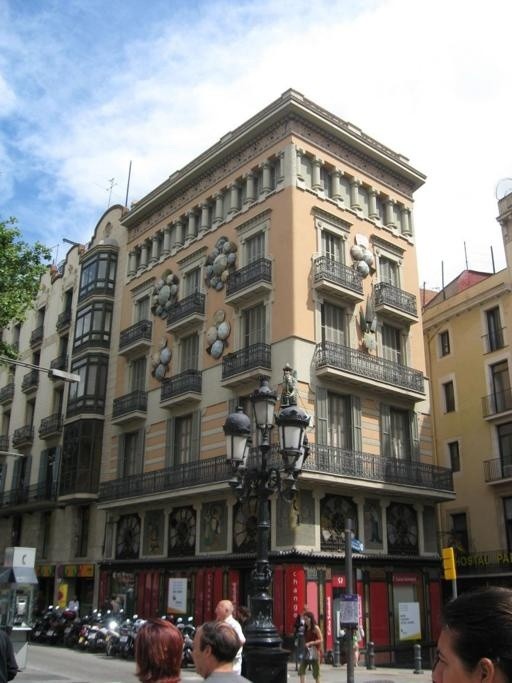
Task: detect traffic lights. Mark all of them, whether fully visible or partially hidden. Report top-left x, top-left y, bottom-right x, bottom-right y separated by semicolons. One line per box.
442;547;456;582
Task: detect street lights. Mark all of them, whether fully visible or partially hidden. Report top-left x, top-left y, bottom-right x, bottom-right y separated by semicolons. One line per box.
217;378;313;682
0;355;84;385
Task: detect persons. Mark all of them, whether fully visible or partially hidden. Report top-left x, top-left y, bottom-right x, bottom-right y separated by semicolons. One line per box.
352;624;366;668
190;619;253;682
214;598;248;675
131;617;185;682
297;610;325;682
429;586;511;683
68;594;80;617
0;629;20;682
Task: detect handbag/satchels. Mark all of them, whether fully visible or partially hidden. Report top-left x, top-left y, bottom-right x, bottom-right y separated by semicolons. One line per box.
305;645;318;665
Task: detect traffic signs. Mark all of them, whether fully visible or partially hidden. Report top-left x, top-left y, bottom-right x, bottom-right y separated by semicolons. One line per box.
351;537;365;553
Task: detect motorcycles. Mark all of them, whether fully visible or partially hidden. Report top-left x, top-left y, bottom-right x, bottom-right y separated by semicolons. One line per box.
28;603;196;671
